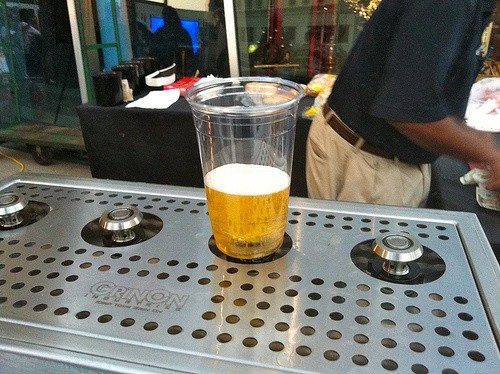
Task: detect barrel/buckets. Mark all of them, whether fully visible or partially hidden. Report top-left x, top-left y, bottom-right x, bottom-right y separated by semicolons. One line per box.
122;60;145;85
93;72;123;107
135;56;158;76
112;65;140;94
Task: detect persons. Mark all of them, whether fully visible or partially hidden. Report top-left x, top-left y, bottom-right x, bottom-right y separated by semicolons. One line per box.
305;0;500;208
150;7;195;74
0;8;42;75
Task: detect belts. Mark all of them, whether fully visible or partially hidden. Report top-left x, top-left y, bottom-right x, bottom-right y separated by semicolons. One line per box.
321;102;419;164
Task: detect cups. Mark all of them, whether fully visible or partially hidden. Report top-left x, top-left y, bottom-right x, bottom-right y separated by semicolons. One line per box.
185;76;306;260
